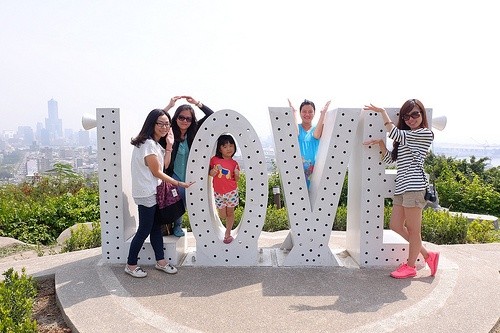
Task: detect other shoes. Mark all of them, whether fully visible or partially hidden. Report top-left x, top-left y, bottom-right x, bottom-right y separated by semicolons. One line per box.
155;262;178;273
174;226;185;237
125;264;148;277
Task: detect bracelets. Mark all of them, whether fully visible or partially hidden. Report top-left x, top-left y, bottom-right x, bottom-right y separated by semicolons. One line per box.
384;121;393;126
165;148;172;152
195;101;200;106
178;181;179;187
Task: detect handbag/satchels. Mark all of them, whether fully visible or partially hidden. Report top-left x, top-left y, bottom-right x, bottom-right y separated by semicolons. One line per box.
156;164;185;225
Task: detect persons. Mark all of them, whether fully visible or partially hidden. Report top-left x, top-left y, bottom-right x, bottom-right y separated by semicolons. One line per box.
208;135;242;243
363;99;441;278
122;109;196;278
158;96;214;237
287;98;332;190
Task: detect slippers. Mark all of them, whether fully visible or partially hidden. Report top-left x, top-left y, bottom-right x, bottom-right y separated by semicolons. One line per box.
224;236;233;244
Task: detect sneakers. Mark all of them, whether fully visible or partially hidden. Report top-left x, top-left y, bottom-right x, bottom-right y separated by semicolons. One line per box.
391;265;418;279
426;252;439;276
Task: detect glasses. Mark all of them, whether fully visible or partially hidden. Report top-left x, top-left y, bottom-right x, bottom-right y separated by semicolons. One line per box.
401;111;422;121
156;123;172;127
178;116;193;122
